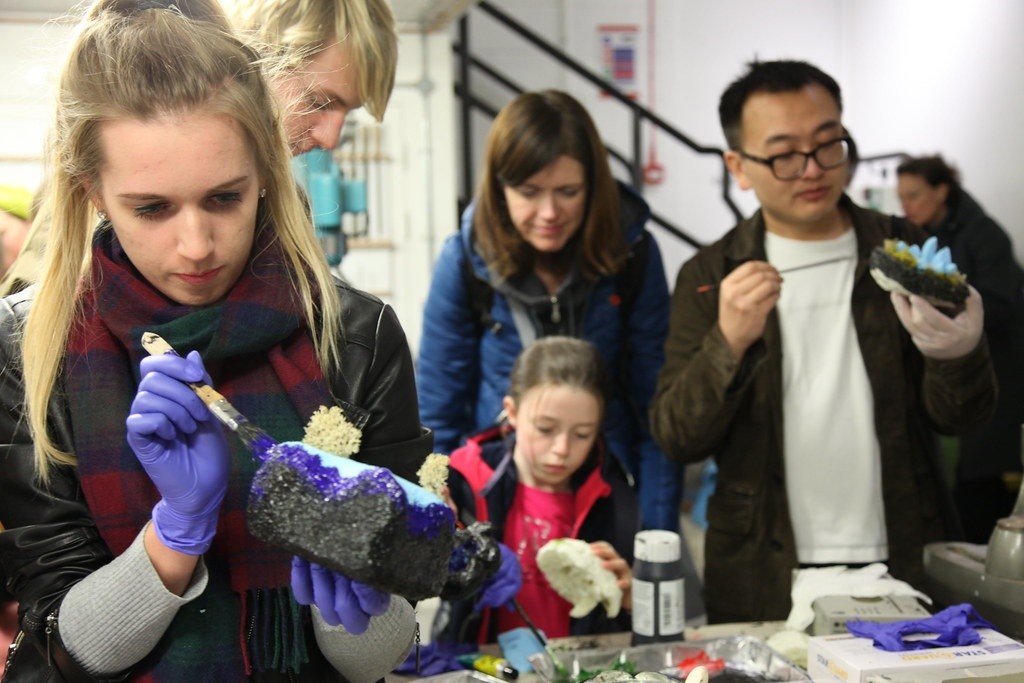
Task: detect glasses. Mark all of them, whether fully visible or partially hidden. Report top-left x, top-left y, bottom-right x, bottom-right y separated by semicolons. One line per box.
739;126;857;183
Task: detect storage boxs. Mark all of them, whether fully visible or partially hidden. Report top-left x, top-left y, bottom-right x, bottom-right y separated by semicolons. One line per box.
807;628;1024;683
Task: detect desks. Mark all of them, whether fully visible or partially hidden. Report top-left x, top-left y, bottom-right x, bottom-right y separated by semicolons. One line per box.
479;621;787;659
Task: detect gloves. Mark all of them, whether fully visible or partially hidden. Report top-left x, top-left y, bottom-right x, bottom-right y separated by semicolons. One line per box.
395;643;481;676
846;603;998;652
291;554;391;635
472;540;523;613
125;351;229;556
890;283;983;361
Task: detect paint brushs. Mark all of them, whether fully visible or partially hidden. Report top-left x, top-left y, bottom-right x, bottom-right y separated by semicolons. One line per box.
696;246;871;293
141;330;278;464
510;595;570;683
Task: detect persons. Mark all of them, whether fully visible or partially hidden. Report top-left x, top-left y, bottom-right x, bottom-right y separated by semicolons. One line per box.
896;157;1024;544
647;61;1000;626
222;0;398;156
415;88;686;535
441;334;644;646
0;0;432;683
